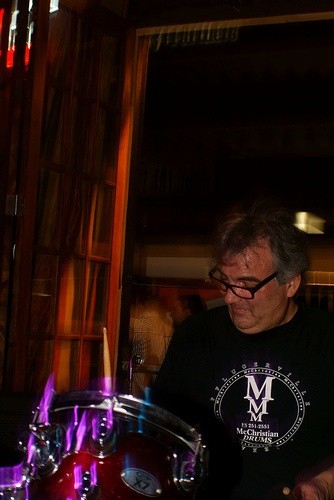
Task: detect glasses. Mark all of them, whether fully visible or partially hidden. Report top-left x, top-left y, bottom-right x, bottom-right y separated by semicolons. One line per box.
208;265;277;300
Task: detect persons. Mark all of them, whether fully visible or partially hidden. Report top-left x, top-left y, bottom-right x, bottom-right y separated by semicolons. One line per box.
149;202;334;500
170;295;207;328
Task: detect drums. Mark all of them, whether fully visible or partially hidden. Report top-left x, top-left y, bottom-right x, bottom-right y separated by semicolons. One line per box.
0;389;208;500
